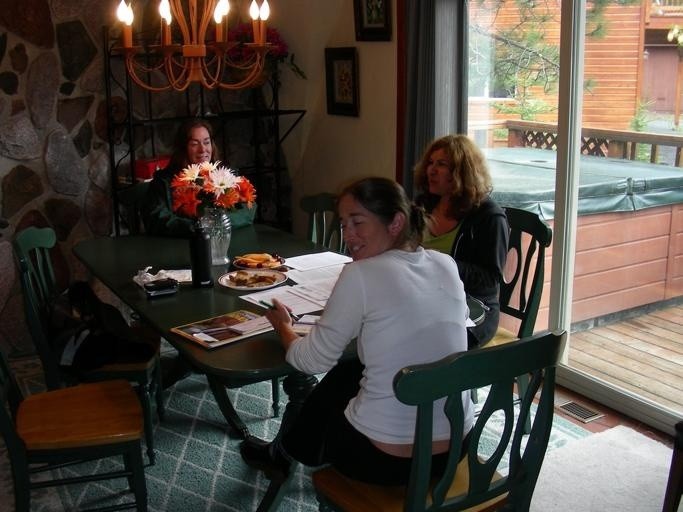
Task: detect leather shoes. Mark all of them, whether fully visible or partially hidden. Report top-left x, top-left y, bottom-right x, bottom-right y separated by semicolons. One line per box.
241;436;290;472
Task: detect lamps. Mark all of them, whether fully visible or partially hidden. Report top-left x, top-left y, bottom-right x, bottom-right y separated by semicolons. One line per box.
114;0;279;93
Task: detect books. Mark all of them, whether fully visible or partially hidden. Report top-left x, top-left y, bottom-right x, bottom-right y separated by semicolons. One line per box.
289;313;321;334
169;308;273;350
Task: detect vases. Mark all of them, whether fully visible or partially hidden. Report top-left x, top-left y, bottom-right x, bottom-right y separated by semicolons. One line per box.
191;205;233;266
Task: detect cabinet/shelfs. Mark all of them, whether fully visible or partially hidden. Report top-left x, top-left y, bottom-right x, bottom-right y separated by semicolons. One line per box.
101;24;307;238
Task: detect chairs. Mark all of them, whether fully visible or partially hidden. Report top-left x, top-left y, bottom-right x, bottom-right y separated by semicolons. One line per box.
11;226;167;466
471;206;552;434
299;191;350;256
0;343;148;511
257;328;567;512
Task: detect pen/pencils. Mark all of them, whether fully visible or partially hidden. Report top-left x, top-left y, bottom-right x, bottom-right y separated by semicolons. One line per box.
259;300;300;321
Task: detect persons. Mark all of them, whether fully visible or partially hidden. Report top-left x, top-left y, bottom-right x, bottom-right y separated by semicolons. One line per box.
237;175;477;485
407;134;510;351
140;118;259;239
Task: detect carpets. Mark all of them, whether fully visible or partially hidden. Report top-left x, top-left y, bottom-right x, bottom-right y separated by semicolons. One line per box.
0;328;683;511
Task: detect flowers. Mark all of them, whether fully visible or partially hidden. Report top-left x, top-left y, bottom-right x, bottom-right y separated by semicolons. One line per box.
204;21;289;66
170;159;257;243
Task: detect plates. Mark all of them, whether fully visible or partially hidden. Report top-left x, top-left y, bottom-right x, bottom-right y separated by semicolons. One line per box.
232;257;286;269
216;269;287;292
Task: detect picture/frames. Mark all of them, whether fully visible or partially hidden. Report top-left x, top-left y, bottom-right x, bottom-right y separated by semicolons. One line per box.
324;47;359;117
352;0;391;41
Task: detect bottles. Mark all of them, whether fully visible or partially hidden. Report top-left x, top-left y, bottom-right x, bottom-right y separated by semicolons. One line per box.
188;227;215;289
